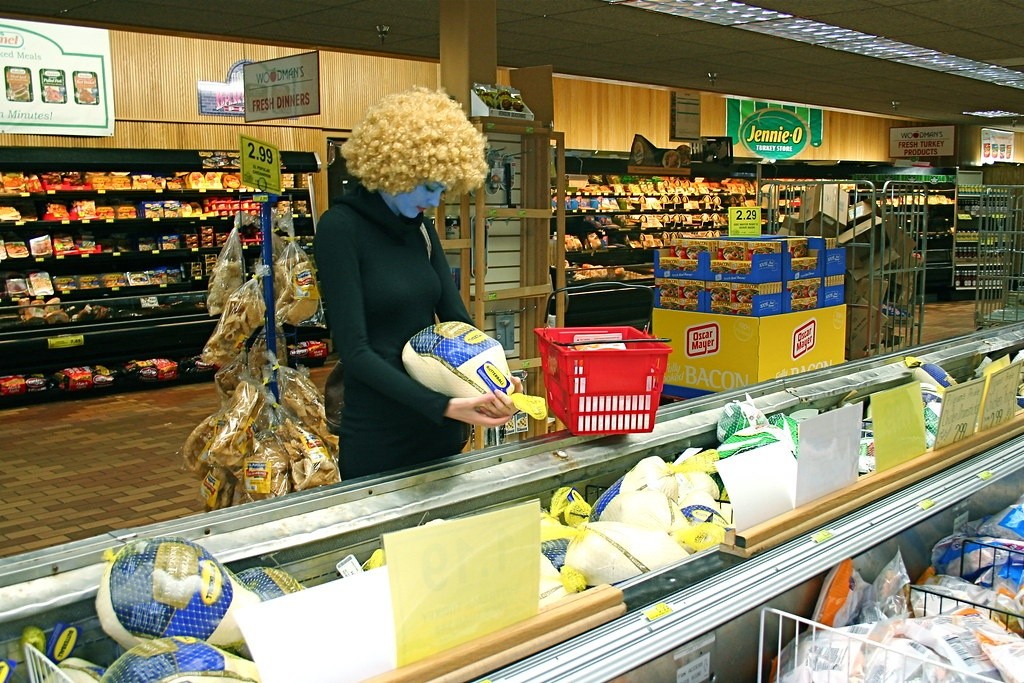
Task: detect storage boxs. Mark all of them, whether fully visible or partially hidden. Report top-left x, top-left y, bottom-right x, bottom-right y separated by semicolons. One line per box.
777;185;920;360
655;234;849;317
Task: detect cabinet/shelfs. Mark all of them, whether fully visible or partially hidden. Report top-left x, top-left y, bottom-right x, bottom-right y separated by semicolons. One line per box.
550;149;855;327
0;145;330;408
853;166;1015;303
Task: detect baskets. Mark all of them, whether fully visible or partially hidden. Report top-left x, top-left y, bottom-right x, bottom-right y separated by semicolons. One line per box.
532;281;673;435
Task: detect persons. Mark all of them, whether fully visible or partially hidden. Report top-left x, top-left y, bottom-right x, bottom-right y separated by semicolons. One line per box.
313;87;525;481
845;200;921;351
